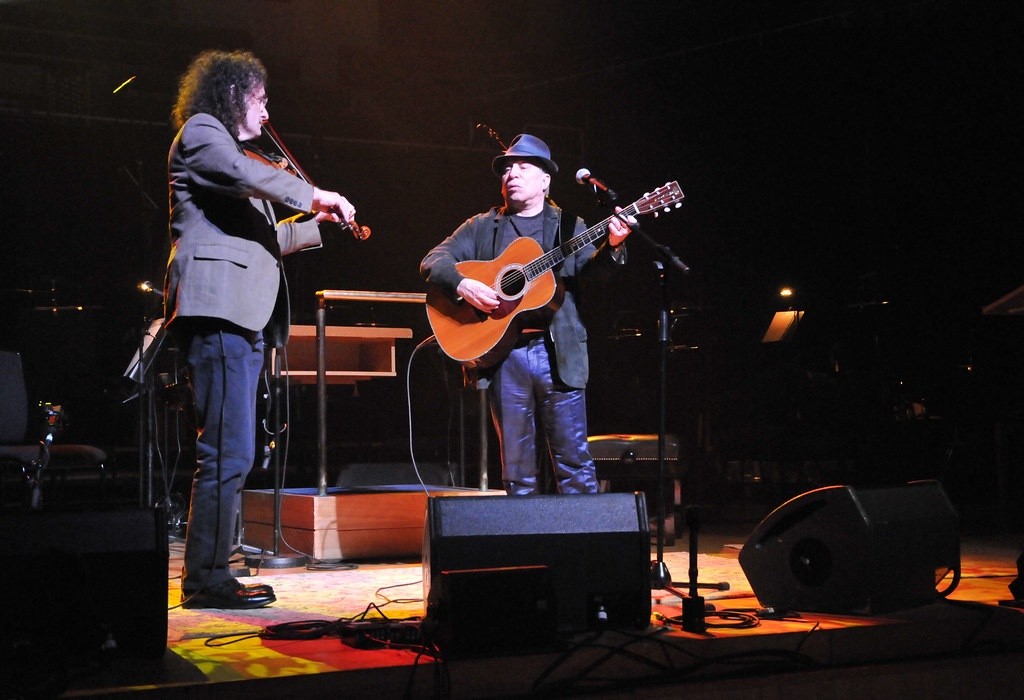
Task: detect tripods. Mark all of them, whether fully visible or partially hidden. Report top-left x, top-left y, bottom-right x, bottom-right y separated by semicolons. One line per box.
594;190;729;613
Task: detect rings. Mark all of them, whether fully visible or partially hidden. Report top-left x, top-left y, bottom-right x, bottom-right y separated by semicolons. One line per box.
618;226;623;231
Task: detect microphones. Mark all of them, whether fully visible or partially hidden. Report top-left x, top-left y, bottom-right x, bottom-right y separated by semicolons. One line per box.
576;169;617;201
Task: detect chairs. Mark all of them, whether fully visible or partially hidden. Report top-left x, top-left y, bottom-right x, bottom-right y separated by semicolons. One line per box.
0;351;107;514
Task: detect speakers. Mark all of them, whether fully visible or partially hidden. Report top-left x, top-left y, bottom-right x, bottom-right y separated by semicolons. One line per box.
585;433;689;527
336;462;460;487
739;478;962;615
423;491;652;656
0;503;169;683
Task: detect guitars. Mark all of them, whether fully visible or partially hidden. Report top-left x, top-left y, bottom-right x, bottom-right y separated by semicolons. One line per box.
424;179;687;368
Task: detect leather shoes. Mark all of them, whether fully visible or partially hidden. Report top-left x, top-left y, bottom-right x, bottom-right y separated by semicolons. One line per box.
233;580;274;593
182;589;277;610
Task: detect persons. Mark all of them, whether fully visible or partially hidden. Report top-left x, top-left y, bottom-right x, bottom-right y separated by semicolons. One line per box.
163;53;355;609
421;134;638;495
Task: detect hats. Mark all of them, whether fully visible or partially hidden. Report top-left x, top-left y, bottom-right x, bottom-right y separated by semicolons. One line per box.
491;134;558;178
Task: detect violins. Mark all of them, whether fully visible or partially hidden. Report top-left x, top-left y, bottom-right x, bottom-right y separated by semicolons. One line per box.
240;142;373;242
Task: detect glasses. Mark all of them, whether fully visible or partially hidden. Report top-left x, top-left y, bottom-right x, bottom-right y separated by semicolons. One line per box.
250;91;268;111
